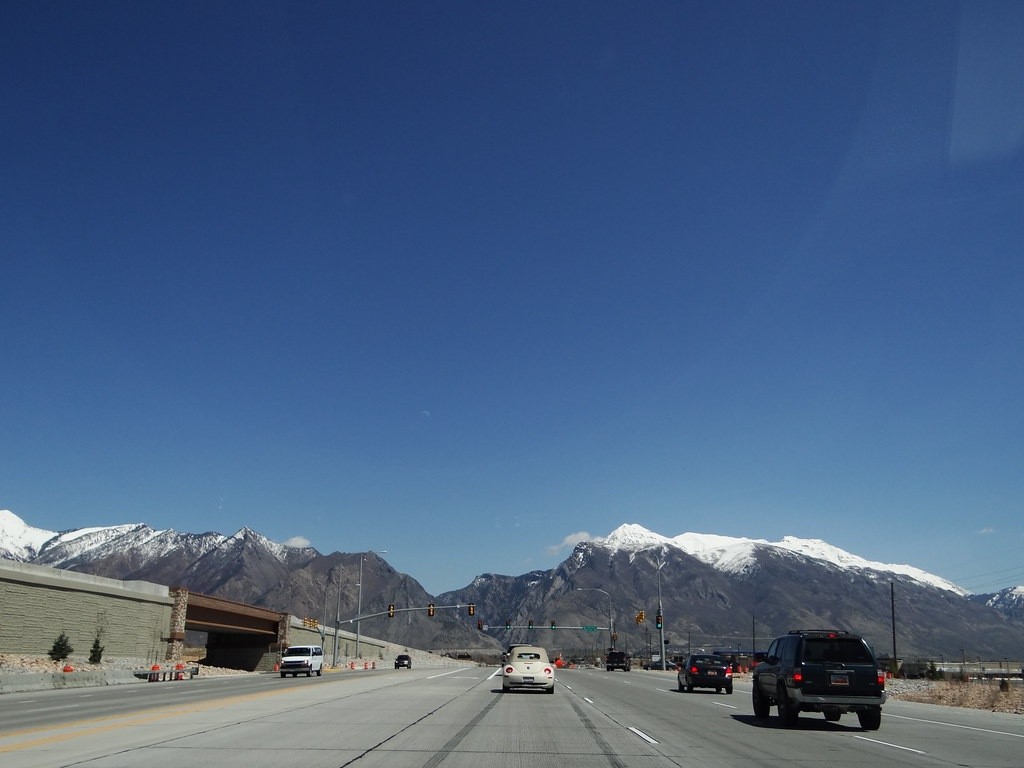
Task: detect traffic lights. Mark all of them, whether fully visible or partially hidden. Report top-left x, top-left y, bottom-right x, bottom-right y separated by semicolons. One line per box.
477;619;483;629
636;611;645;624
505;620;510;629
528;619;534;629
655;616;662;630
551;619;555;630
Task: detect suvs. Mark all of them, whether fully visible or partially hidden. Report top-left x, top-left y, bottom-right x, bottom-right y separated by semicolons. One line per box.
606;650;631;671
280;645;323;676
750;628;888;732
395;654;412;669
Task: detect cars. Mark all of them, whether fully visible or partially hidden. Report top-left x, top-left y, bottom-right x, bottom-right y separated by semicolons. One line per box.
500;644;531;668
643;658;677;672
677;653;733;695
502;645;555;694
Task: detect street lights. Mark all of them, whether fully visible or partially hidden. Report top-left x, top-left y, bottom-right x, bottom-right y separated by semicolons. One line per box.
355;550;387;659
321;581;362;654
577;586;614;649
